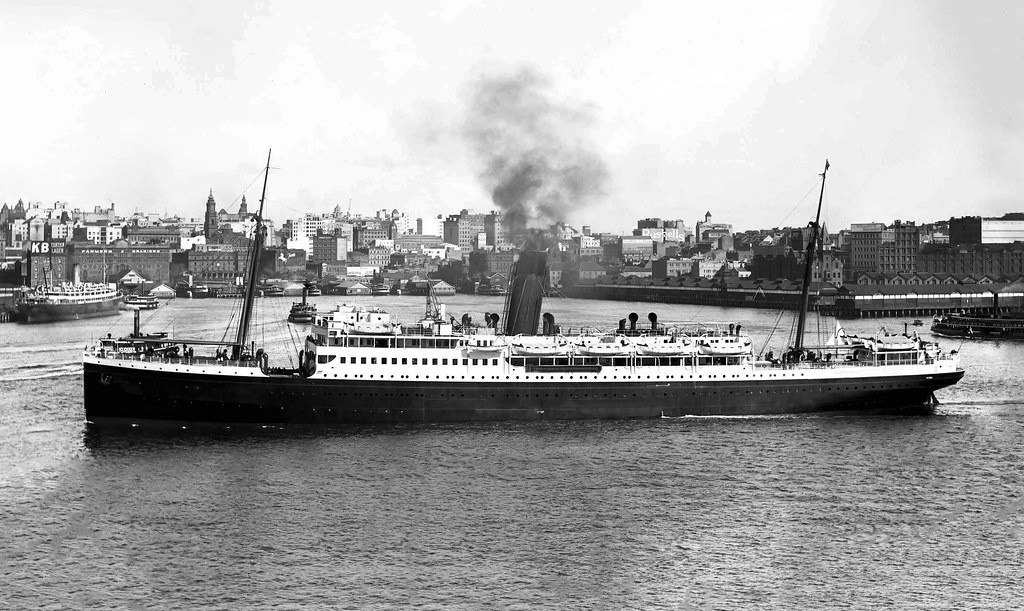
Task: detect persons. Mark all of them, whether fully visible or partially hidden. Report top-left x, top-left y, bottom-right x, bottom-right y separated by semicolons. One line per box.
220;349;229;360
256;349;263;369
766;347;832;367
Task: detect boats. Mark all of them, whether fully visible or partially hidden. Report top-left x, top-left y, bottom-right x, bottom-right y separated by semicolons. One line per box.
15;238;125;324
574;343;632;355
477;284;504;296
700;343;751;355
465;344;507;354
287;287;317;323
511;343;571;356
124;281;160;310
81;147;966;426
637;343;694;355
928;308;1024;339
